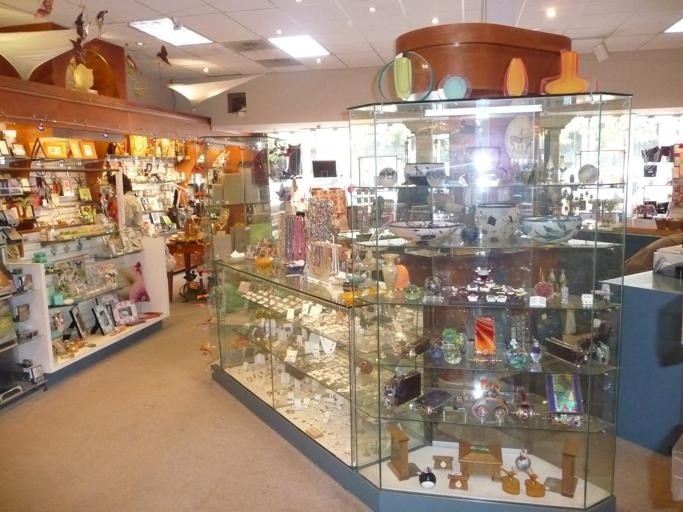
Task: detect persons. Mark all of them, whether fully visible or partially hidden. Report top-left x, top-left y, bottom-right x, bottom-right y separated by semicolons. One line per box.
107;173;144;229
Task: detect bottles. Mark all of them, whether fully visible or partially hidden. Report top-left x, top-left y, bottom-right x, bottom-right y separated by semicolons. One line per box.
381;253;400;299
548;268;569;306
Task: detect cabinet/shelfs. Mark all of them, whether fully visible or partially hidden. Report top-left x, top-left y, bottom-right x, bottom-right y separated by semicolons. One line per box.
0;119;168;399
155;135;265;275
